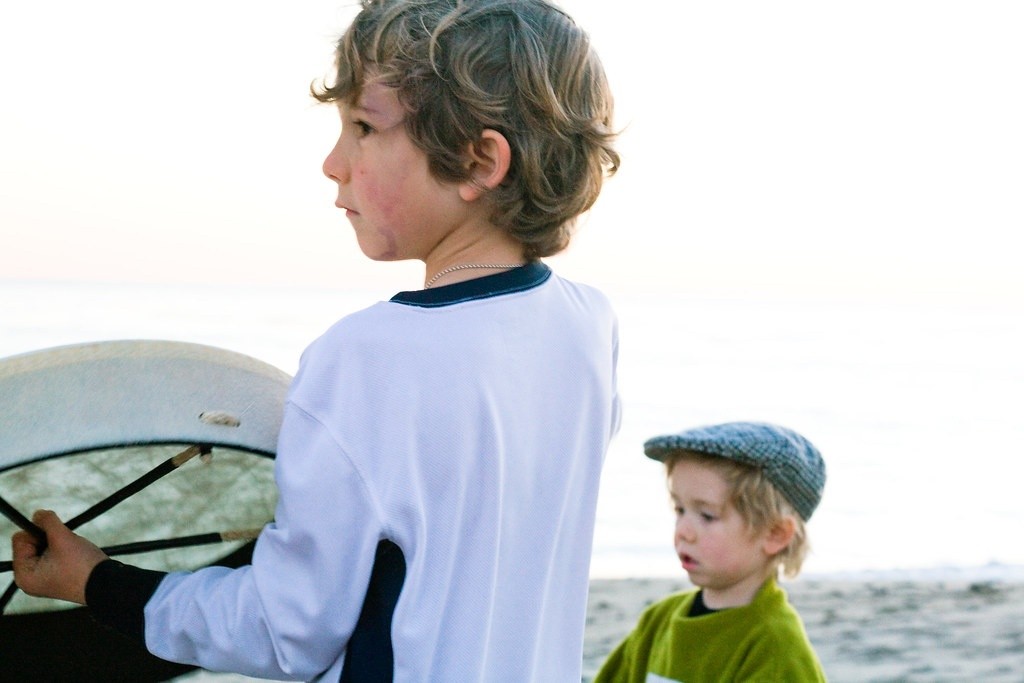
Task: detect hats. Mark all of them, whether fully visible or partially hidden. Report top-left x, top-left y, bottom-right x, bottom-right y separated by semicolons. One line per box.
644;422;827;522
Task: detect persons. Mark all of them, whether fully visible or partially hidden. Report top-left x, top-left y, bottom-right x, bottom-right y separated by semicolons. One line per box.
593;421;827;683
11;0;622;683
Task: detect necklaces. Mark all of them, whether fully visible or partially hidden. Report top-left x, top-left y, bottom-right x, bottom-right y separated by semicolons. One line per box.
423;263;524;289
